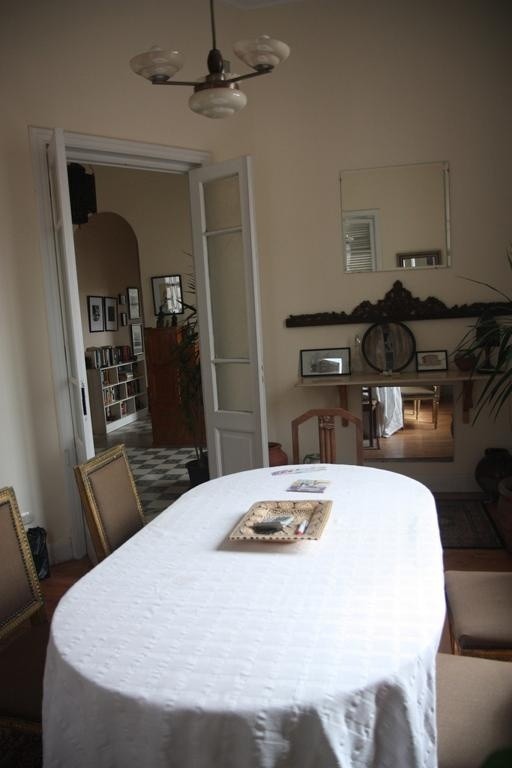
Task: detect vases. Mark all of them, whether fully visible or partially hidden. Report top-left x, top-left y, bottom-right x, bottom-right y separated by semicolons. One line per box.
473;447;511;496
266;441;288;467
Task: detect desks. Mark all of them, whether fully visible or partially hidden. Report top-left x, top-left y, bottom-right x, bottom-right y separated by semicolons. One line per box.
39;462;444;768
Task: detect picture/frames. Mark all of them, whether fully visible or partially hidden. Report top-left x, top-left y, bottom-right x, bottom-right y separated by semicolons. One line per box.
300;347;352;376
415;349;448;372
88;286;145;356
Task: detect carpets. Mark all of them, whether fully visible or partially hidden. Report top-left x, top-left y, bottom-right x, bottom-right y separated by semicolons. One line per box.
433;491;506;550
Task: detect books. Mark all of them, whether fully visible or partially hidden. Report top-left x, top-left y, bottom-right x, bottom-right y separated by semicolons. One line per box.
85;344;152;432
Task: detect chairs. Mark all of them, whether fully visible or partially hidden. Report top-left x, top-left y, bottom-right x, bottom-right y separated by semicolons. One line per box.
71;441;151;563
290;406;363;467
361;387;381;450
399;387;440;432
2;486;51;768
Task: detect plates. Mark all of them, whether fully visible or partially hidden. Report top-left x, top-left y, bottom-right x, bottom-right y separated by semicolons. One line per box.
228;498;332;542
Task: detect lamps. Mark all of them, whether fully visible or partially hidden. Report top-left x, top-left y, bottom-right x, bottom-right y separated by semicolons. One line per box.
127;1;293;120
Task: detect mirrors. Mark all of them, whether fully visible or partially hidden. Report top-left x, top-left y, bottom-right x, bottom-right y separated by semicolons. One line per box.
151;275;184;316
339;162;449;275
393;248;443;270
360;387;453;462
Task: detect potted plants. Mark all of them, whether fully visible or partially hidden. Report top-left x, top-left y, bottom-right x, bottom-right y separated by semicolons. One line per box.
438;248;511;533
166;280;220;481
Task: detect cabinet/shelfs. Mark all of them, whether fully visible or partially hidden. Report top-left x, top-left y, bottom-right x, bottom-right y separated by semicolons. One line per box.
143;325;207;447
85;355;152;435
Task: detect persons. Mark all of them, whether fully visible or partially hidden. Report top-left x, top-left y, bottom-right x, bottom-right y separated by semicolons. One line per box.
93;308;100;320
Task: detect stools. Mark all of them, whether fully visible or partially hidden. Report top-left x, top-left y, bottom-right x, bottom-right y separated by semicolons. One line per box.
435;648;512;767
445;568;511;657
